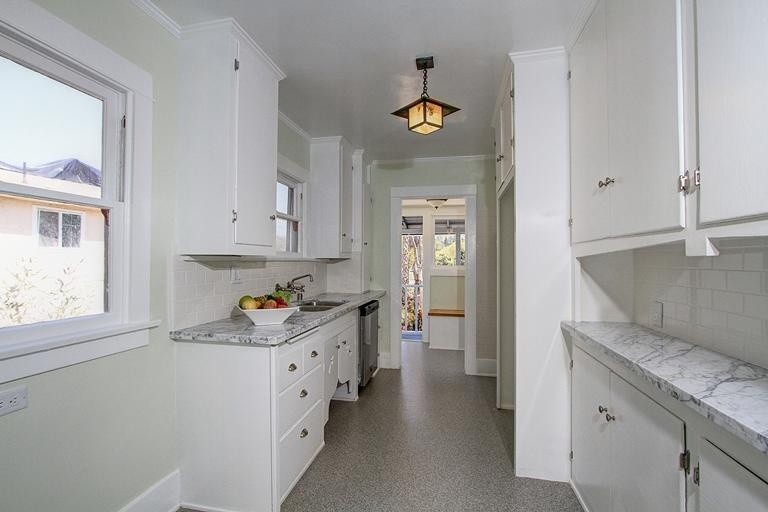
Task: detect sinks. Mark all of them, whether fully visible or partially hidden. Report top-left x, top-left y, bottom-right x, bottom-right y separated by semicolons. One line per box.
299;299;349;306
299;306;334;312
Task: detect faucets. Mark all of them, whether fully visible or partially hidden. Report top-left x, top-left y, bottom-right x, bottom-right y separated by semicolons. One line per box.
290;274;314;294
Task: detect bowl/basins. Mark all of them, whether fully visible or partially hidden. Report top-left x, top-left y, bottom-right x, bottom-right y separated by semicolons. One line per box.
235;301;299;326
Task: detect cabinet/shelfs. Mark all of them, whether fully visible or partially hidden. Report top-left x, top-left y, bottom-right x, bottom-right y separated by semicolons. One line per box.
491;58;513;199
695;0;768;229
173;326;328;512
326;183;375;295
569;0;686;245
569;345;688;512
174;18;288;261
309;136;354;261
694;439;768;512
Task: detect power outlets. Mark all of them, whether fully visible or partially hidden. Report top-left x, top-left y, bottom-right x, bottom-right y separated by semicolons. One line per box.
0;384;29;417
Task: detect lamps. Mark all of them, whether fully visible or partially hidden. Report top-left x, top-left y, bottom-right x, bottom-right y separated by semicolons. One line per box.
390;55;462;136
